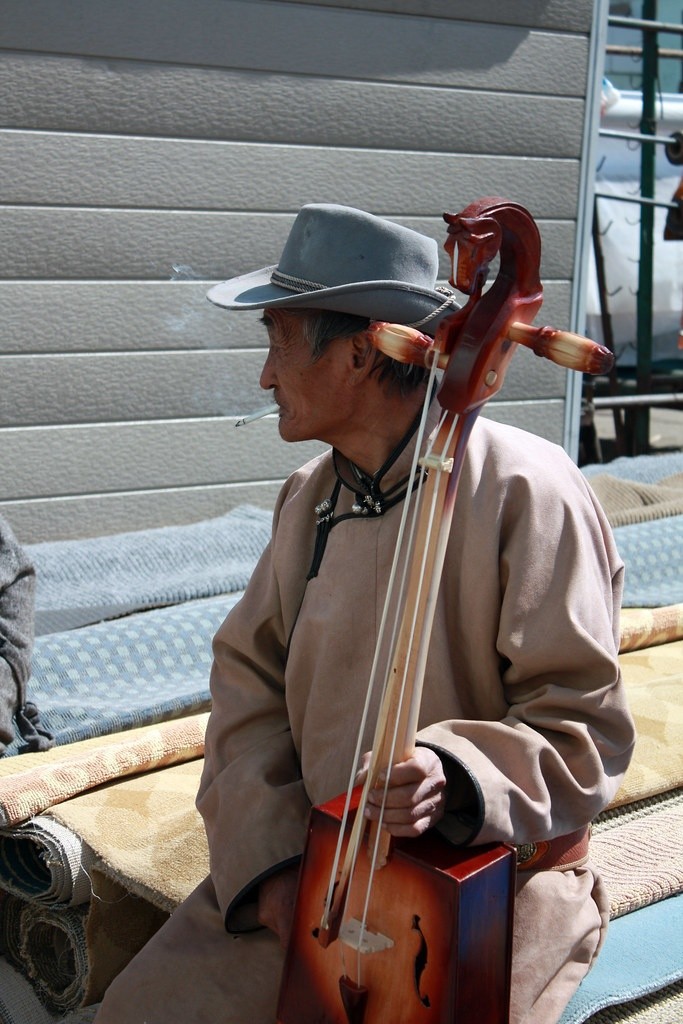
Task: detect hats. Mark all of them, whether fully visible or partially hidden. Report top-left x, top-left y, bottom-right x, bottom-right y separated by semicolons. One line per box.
205;203;464;334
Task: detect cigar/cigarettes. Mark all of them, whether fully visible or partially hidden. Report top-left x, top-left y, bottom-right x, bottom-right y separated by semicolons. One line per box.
235;403;281;427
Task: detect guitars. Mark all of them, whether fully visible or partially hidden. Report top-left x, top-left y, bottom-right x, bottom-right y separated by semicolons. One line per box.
269;195;618;1024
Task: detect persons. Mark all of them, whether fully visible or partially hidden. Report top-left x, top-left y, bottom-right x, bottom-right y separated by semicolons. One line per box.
90;203;636;1024
